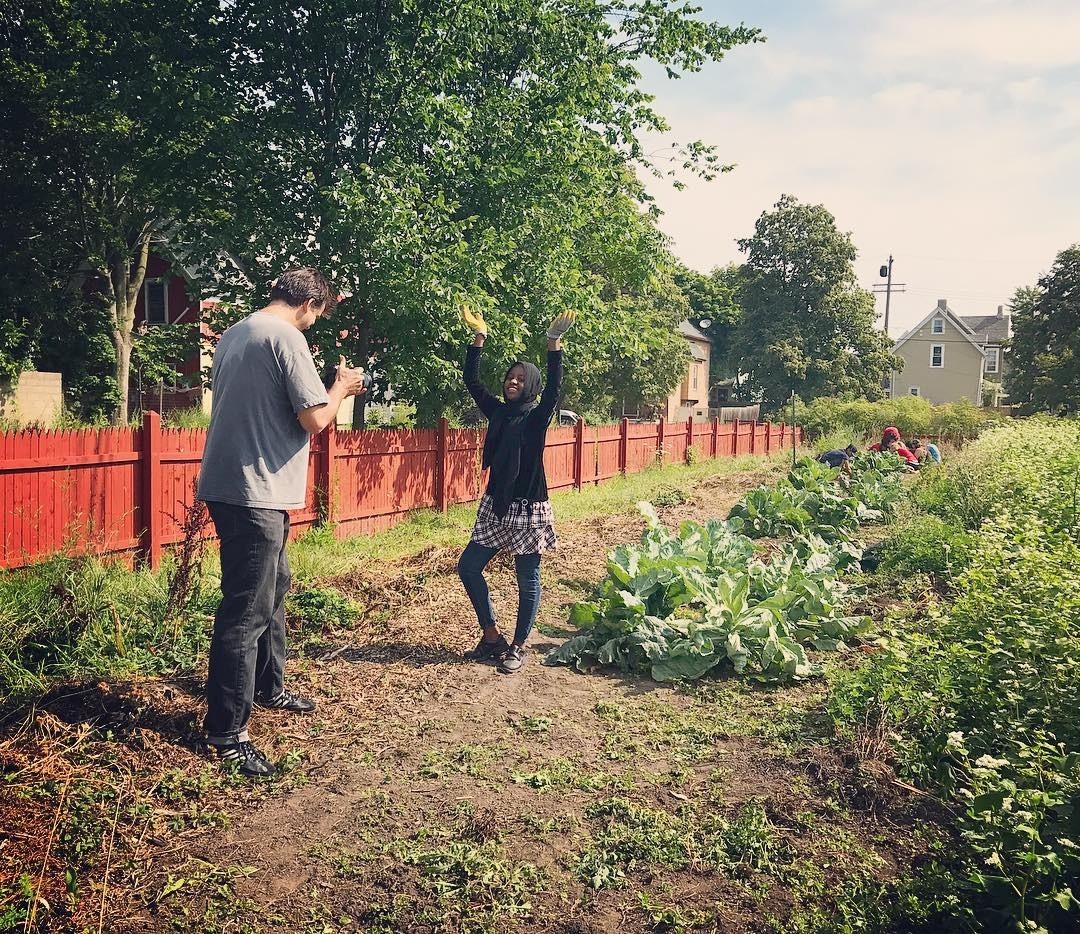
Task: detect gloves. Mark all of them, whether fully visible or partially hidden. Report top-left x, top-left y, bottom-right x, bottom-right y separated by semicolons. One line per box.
461;304;487;339
545;309;575;339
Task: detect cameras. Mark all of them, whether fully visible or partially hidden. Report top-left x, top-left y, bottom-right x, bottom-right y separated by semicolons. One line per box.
325;364;372;393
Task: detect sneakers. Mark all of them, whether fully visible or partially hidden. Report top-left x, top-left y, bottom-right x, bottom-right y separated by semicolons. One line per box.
257;688;316;712
500;643;526;673
465;634;509;662
213;741;277;777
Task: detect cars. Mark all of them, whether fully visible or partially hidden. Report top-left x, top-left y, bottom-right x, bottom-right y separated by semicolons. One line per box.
559;409;580;426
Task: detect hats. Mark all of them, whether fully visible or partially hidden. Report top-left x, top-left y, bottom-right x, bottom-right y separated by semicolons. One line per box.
848;444;857;454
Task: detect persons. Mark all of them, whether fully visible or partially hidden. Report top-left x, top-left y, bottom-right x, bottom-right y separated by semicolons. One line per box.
191;268;367;778
458;303;577;675
818;427;941;478
